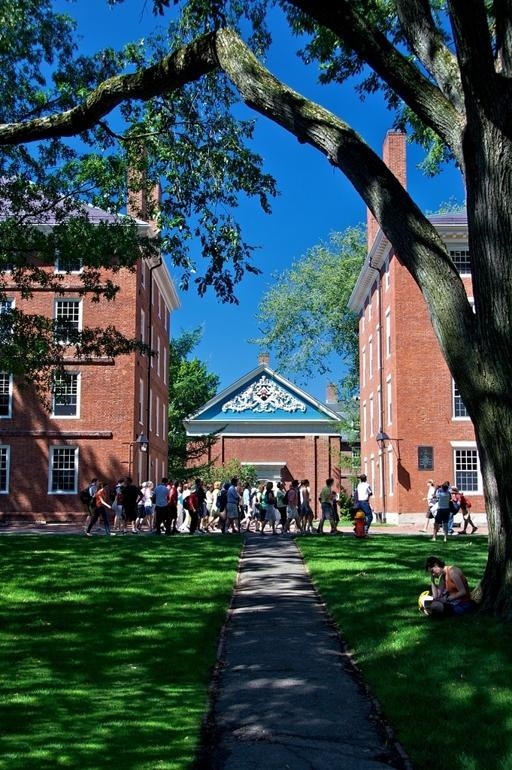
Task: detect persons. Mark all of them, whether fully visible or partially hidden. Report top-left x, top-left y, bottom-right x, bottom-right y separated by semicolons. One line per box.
422;556;469;620
85;475;371;533
419;480;478;541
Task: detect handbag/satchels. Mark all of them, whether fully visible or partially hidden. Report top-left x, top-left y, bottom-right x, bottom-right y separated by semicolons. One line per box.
111;498;118;515
282;496;288;505
90;495;96;509
350;501;360;520
183;496;190;509
266;495;277;504
79;489;92;505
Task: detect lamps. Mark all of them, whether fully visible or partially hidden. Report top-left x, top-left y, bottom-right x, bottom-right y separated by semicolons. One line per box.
136;430;149;452
375;426;404;452
